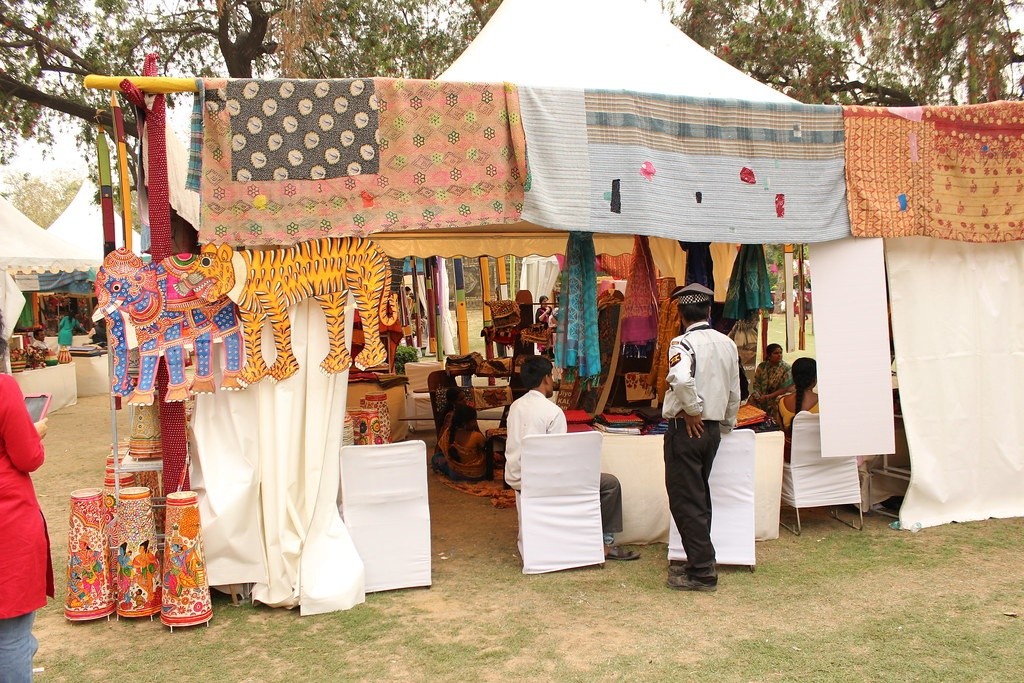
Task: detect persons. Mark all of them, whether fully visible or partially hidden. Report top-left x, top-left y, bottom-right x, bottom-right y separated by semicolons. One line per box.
662;283;741;592
747;343;793;431
88;316;107;345
535;295;560;361
504;356;641;560
738;356;749;401
432;387;488;482
0;308;55;683
405;286;412;310
776;357;819;462
57;310;89;350
32;328;49;351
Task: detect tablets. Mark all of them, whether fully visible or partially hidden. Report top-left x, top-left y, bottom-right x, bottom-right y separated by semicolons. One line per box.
24;393;52;423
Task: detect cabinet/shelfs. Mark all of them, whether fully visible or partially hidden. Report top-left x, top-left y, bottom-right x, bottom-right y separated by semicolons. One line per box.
868;387;913;520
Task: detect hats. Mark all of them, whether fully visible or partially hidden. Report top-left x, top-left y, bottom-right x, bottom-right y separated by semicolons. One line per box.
670;283;713;305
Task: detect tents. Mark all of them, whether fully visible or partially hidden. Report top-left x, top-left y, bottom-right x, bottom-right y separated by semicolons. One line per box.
435;0;804;351
0;179;146;293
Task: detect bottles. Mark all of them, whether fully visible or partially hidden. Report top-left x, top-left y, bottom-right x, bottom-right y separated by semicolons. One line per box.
44;349;58;365
58;346;72;364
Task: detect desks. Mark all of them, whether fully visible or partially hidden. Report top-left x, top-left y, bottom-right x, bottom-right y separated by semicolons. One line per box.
43;334;93;352
12;361;78;415
589;413;786;546
72;352;110;398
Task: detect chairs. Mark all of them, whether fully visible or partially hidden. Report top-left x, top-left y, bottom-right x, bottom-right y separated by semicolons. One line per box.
339;296;870;596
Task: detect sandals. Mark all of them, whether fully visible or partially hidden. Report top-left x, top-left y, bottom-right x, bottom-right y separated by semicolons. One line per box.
606;546;640;560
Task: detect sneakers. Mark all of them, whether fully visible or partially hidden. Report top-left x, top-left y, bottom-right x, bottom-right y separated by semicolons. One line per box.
668;575;716;591
669;565;687;576
